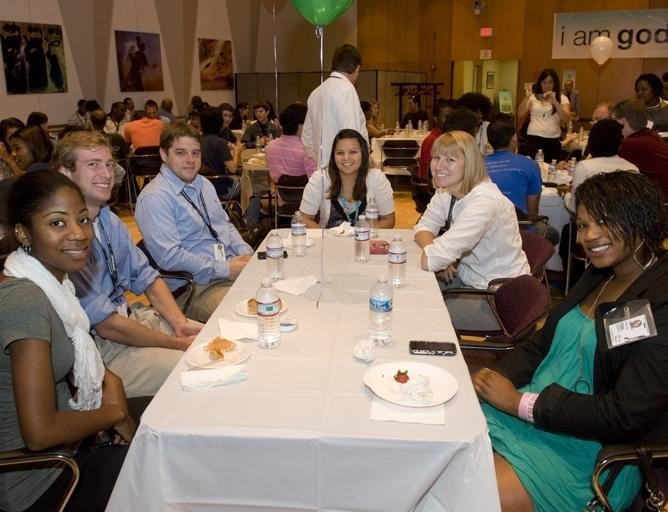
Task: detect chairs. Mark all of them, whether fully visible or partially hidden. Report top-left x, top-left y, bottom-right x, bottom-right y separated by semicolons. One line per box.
1;138;667;512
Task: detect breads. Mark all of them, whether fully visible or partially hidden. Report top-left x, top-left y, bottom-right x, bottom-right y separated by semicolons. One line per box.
247;298;258;316
206;336;237;362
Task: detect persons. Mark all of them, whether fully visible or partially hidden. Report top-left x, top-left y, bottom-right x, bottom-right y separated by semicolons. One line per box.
413;69;667;512
301;44;371;168
0;68;668;512
243;97;426;229
124;36;148;91
46;40;63;88
2;95;254;512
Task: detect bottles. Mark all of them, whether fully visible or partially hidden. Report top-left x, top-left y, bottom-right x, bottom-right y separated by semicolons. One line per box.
290;211;308;257
394;117;429;138
267;133;272;144
364;196;380;238
566;120;582;144
255;135;261;152
368;274;394;346
241;120;246;131
265;230;283;281
386;233;409;288
353;213;371;263
254;277;282;349
532;147;578;186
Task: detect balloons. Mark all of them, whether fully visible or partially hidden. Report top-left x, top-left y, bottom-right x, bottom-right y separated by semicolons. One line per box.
292;0;353;27
590;36;613;67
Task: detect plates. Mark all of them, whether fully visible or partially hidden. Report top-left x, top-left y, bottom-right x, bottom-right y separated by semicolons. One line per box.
324;228;352;237
185;340;251;368
360;359;459;408
234;297;289;317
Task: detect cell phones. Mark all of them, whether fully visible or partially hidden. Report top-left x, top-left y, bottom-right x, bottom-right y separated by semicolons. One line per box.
409;340;457;356
257;251;288;260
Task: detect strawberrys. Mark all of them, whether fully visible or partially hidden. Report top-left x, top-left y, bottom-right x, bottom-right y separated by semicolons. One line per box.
394;369;408;383
